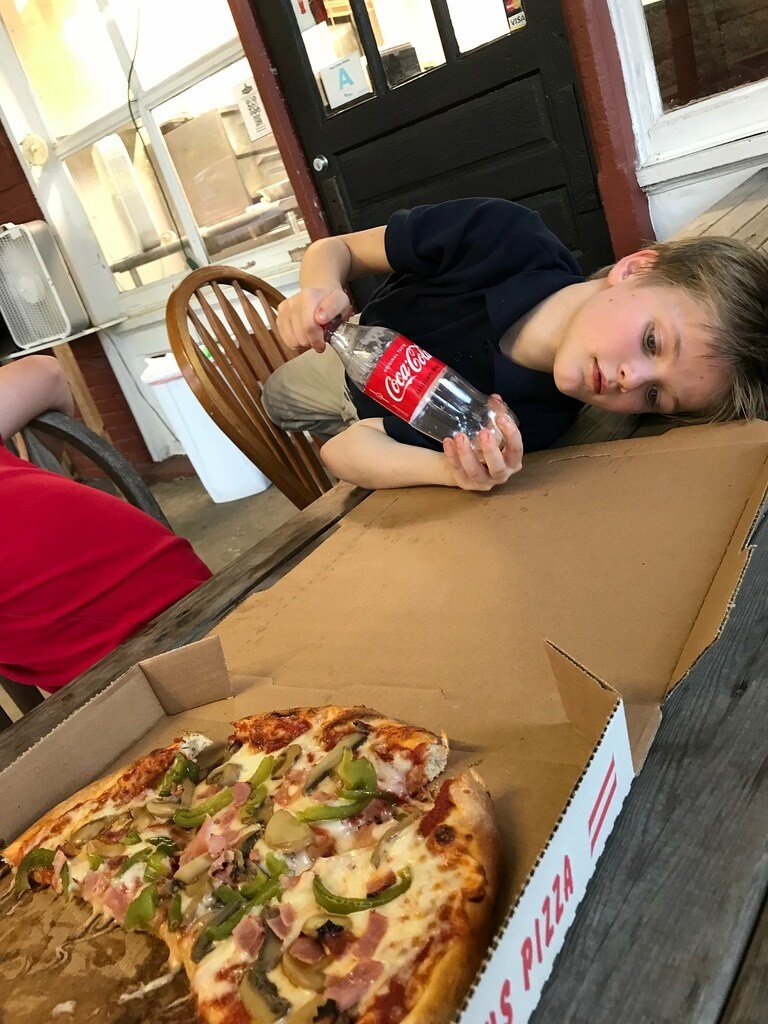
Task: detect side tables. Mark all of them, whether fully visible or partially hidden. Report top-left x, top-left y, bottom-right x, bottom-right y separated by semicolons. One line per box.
1;317;132;482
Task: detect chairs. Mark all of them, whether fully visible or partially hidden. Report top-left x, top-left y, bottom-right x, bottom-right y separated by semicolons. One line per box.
165;264;340;512
0;408;177;731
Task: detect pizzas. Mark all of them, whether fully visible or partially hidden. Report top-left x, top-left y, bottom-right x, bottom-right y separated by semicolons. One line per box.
0;704;505;1024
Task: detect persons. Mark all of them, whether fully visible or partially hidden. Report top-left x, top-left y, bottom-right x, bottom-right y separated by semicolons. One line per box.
0;356;214;695
264;198;767;492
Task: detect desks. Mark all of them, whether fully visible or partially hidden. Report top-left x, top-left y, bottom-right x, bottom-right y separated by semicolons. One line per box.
0;420;768;1022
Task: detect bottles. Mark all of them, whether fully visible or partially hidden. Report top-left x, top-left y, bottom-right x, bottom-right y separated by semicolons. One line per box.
321;313;518;465
